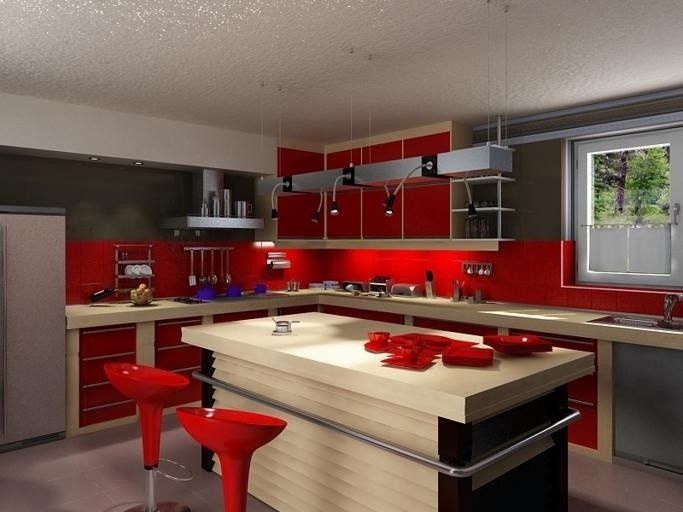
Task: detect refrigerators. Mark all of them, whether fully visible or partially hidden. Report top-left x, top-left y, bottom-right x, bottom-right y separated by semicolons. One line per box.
0;206;66;453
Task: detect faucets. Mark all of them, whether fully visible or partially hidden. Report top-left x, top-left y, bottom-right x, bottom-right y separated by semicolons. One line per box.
661;295;683;324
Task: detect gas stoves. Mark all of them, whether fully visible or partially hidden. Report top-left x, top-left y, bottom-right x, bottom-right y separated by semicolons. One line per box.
172;296;210;304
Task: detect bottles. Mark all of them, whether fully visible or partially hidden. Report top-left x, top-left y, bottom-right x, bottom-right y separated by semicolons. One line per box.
88;288;119;302
424;271;436;298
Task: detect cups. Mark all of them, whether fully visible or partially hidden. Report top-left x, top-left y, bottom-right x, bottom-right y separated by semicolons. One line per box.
368;332;392;343
290;321;302;335
401;345;418;364
276;320;290;332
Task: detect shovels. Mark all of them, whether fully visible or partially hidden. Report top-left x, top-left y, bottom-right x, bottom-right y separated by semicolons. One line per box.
188;249;197;286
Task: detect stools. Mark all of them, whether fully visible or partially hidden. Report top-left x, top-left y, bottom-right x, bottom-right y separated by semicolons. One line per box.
176;406;288;512
104;361;191;512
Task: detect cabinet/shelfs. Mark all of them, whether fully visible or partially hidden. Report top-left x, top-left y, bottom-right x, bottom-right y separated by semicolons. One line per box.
136;315;203;421
65;323;136;439
509;329;614;465
253;121;516;252
114;245;156;297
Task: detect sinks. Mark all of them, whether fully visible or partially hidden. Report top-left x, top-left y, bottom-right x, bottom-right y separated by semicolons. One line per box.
660;319;683;331
588;314;658;327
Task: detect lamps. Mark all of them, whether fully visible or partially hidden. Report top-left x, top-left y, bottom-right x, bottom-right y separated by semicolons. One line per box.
259;0;515;220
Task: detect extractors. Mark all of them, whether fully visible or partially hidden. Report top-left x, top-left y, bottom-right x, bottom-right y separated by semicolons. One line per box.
161;168;265;230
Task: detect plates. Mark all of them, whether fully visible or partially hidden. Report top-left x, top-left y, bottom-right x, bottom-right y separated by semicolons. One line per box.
140;264;153;274
124;265;132;276
132;265;140;276
482;334;553;355
442;346;494;368
273;330;292;335
381;353;436;370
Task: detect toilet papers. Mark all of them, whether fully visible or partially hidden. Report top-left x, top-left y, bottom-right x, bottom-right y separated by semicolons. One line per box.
271;261;290;269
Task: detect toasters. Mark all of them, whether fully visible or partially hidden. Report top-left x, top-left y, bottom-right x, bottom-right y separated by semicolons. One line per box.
389;283;425;297
